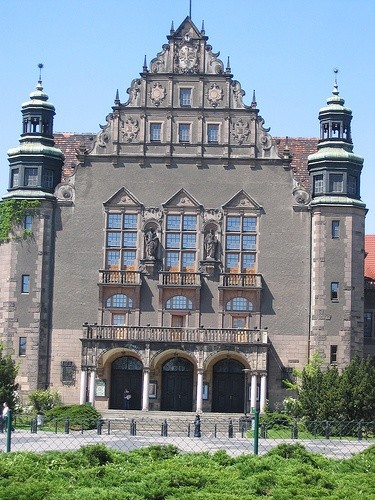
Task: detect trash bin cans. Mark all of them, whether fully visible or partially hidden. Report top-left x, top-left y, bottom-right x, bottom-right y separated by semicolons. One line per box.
36;411;45;425
238;415;251;431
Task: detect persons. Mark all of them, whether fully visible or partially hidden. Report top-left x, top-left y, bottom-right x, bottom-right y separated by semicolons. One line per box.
203;229;218;260
3;402;16;434
123;388;132;409
144;226;159;259
